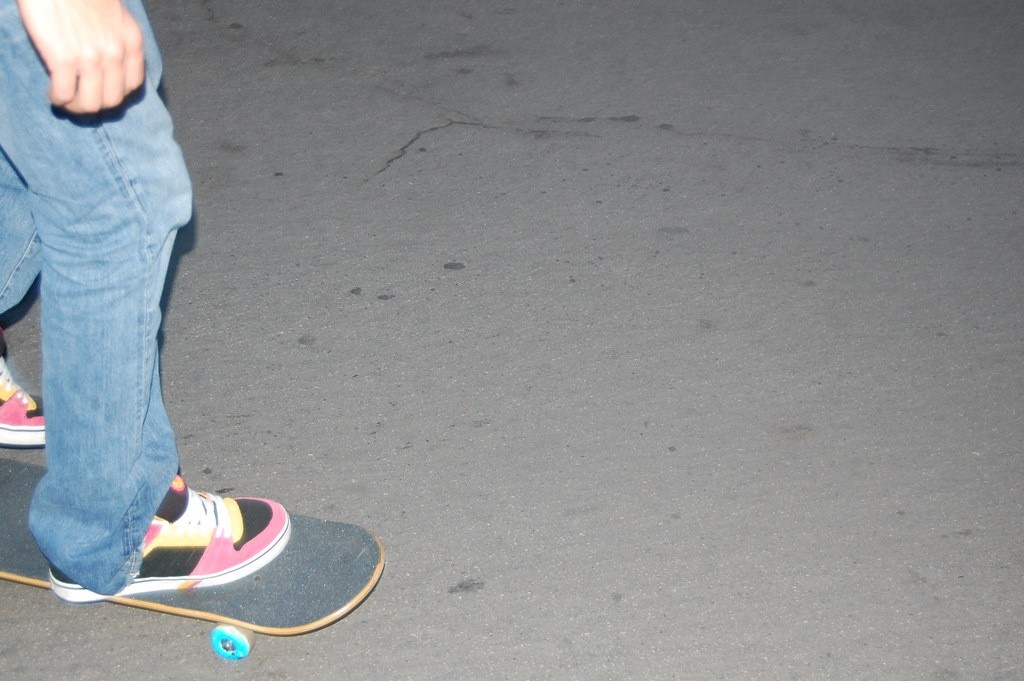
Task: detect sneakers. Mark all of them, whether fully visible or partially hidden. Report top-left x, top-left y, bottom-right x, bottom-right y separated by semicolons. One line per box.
1;358;46;448
46;476;291;606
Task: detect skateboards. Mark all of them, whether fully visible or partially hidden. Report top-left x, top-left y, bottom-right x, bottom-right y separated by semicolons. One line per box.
0;458;385;659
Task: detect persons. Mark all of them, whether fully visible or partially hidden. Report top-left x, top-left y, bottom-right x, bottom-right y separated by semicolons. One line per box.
0;0;293;603
